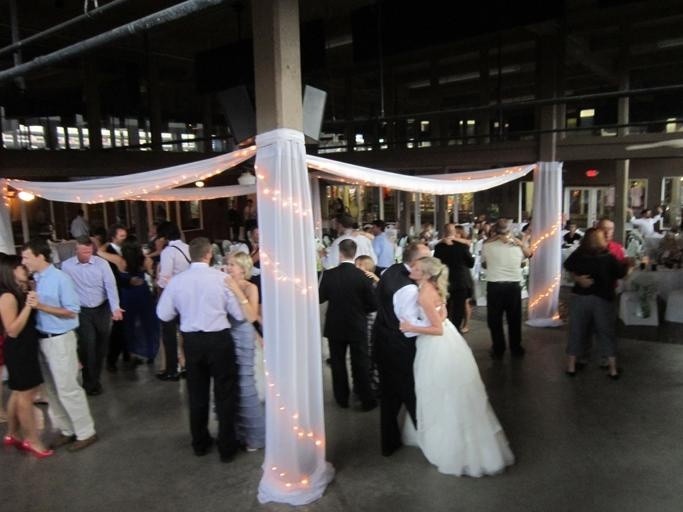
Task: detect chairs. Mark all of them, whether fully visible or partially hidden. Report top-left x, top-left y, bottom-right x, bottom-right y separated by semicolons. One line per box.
383;223;682;328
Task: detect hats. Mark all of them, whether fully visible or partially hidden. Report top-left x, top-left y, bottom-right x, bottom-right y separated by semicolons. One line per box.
493;218;513;235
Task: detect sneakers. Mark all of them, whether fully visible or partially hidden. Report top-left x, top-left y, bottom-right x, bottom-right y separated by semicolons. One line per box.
82;380;102;395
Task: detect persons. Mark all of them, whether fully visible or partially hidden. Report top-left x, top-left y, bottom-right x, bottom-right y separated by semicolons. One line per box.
398;256;515;478
369;242;448;457
221;250;266;453
21;237;99;452
0;253;56;458
1;184;682;425
155;236;248;462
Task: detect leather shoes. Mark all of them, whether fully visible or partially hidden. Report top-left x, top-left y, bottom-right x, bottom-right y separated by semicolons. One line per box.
65;434;97;452
105;359;117;373
155;371;180;381
48;434;75;450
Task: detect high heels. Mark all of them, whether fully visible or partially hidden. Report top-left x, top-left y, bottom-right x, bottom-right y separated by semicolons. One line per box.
20;438;54;459
2;434;22;453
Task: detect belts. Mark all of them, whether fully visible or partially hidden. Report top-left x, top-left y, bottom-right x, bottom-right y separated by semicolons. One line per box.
36;333;63;338
